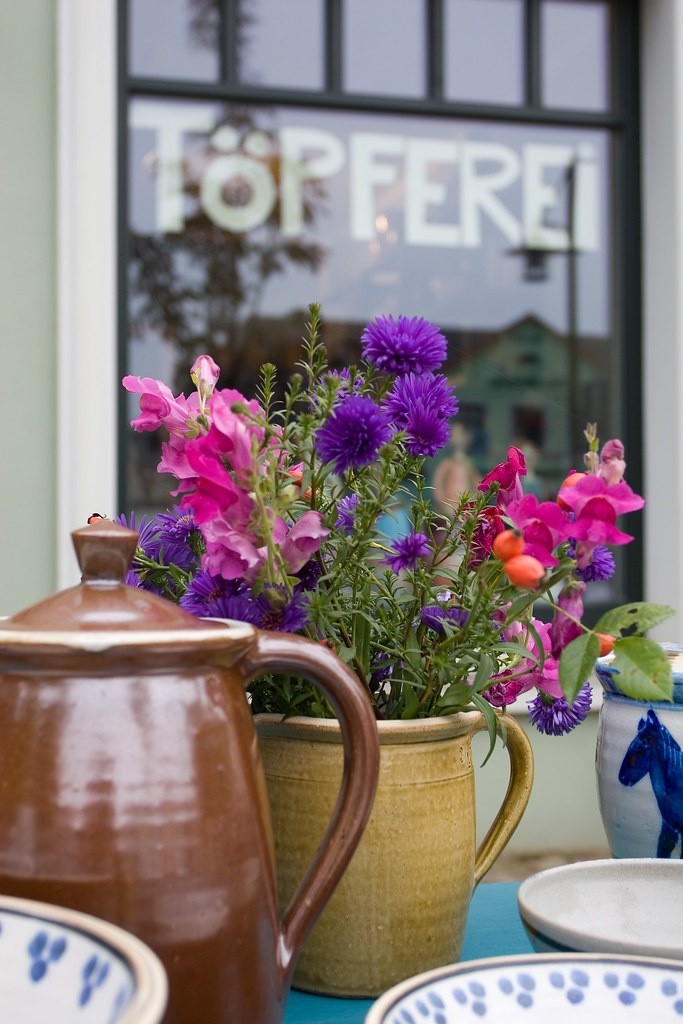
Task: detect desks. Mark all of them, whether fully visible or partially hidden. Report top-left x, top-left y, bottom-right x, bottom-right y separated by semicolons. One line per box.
280;878;565;1024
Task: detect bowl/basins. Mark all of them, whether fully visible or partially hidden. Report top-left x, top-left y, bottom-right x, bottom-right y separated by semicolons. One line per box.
1;894;171;1021
366;955;682;1014
516;857;683;967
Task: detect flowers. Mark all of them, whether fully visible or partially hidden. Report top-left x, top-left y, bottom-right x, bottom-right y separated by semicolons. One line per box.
79;294;678;770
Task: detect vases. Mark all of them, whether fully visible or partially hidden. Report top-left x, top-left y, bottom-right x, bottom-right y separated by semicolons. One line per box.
251;704;538;1006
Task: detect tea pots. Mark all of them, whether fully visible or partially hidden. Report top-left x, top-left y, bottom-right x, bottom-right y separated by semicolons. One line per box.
1;518;379;1023
